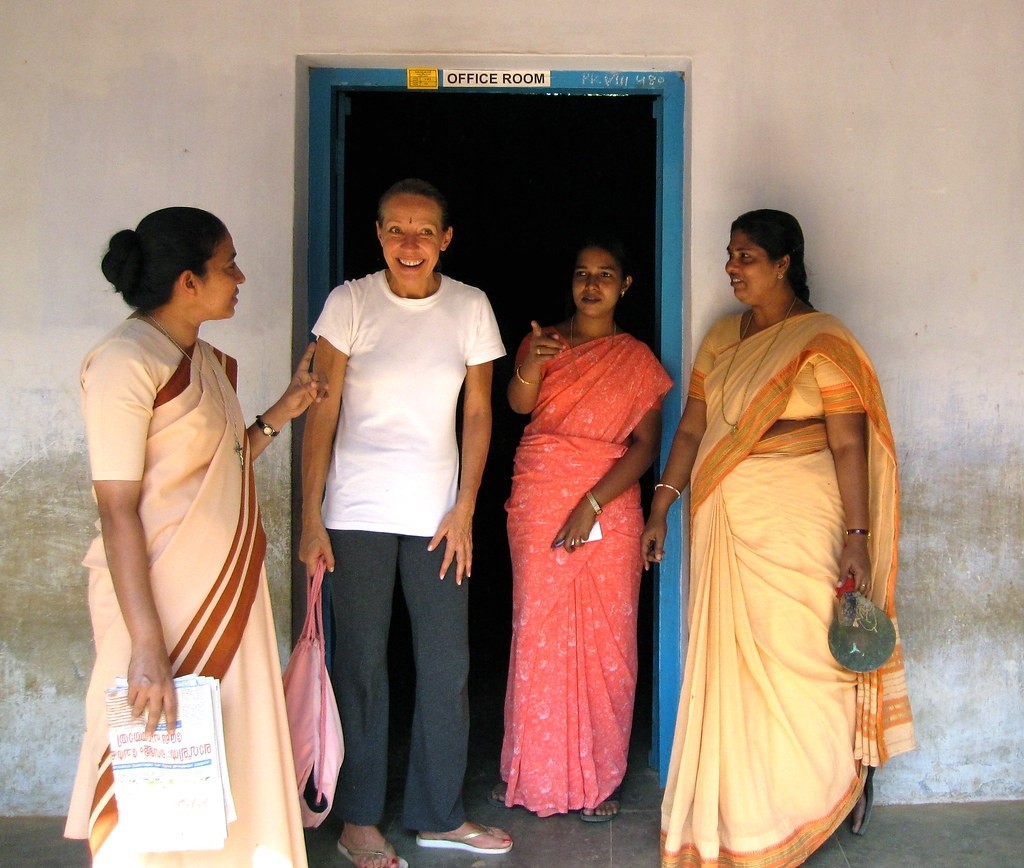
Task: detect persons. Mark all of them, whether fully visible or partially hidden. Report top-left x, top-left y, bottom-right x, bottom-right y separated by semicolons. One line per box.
636;210;912;868
492;233;675;822
63;205;330;868
297;179;514;868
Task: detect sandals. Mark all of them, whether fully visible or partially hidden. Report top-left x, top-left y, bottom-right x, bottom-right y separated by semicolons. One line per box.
486;781;522;808
580;787;622;822
852;765;877;835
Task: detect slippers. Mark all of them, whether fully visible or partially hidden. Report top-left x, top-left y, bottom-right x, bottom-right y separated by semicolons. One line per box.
337;836;408;868
416;823;513;854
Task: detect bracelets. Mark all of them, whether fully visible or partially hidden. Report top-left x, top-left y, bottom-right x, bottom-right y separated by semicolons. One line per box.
846;529;872;537
584;489;602;515
654;482;682;499
517;363;544;385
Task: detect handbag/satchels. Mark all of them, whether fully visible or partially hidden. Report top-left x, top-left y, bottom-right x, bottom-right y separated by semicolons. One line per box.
283;553;346;829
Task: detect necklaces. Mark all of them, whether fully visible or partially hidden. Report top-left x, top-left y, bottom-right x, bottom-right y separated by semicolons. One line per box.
720;293;797;426
148;313;245;467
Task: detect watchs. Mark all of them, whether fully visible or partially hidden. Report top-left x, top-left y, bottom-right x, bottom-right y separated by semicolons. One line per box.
254;414;280;438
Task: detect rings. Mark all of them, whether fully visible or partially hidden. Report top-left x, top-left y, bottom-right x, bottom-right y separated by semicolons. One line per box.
537;346;541;354
861;584;865;587
